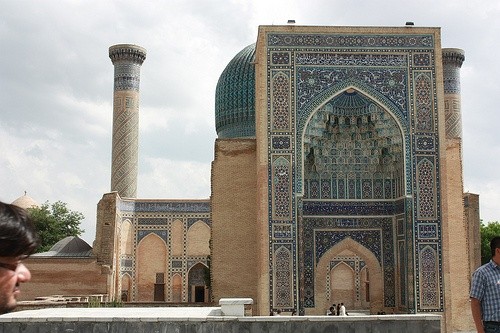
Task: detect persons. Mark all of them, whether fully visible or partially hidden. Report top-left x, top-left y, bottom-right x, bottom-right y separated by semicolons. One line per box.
469;236;500;333
275;310;282;316
328;303;347;316
0;201;40;315
